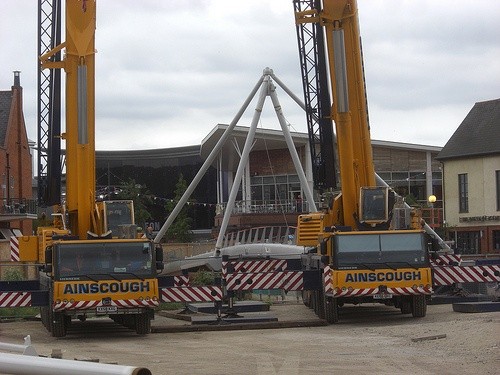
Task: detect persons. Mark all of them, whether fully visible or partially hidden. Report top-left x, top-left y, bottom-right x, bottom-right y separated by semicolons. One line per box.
147;227;153;240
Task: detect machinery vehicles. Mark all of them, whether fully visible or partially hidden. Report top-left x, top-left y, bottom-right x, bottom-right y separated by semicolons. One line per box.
293;0;432;325
3;2;165;337
